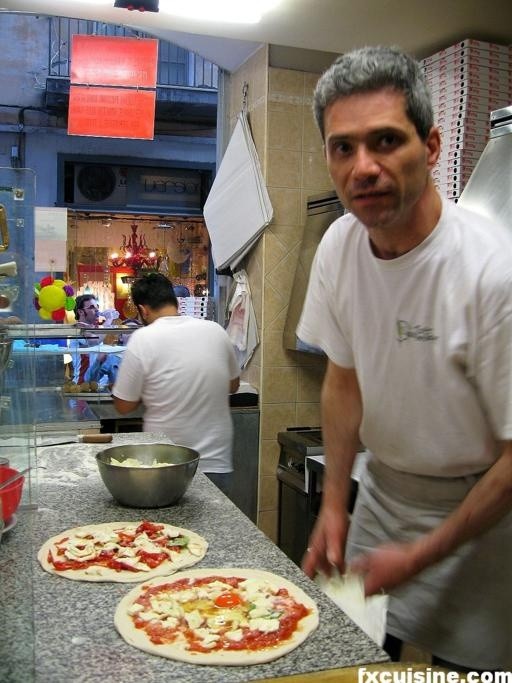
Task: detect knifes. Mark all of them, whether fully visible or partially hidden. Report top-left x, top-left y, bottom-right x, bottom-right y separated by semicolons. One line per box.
31;432;113;448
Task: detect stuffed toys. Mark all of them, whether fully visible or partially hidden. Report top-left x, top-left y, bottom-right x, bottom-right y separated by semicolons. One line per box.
33;277;75;323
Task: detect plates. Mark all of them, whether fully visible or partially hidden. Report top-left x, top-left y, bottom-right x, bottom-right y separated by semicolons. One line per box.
1;514;18;535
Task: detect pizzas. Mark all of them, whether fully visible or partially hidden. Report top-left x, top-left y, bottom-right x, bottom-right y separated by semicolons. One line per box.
113;567;320;667
37;520;209;584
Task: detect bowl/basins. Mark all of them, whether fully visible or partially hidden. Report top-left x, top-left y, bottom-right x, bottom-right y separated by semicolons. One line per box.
0;465;26;522
95;442;201;508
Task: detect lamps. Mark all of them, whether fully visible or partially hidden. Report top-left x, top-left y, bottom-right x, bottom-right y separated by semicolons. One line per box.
109;221;158;274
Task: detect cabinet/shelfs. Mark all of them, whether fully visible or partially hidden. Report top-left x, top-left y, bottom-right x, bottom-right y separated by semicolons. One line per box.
0;315;148;432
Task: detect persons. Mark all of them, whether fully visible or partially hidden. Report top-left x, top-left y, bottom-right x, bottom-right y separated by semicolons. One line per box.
83;319;142;387
70;293;113;383
111;272;243;499
295;43;512;681
